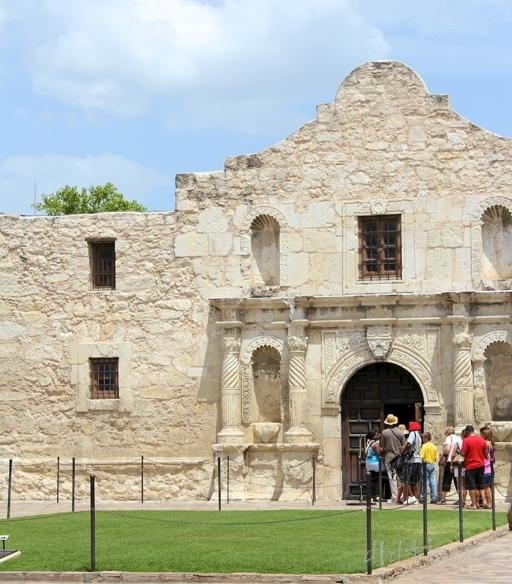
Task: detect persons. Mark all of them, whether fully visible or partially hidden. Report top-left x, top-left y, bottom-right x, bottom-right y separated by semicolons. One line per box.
504;467;511;531
362;412;495;511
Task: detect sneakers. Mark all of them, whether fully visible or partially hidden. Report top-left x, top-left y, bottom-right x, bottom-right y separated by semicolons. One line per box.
370;497;491;509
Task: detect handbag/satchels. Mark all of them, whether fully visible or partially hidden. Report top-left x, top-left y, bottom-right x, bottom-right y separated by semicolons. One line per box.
439;455;446;466
402;442;415;459
390;455;403;472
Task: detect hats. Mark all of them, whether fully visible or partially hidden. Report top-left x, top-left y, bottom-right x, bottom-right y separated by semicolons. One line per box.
384;414;399;425
397;424;410;435
